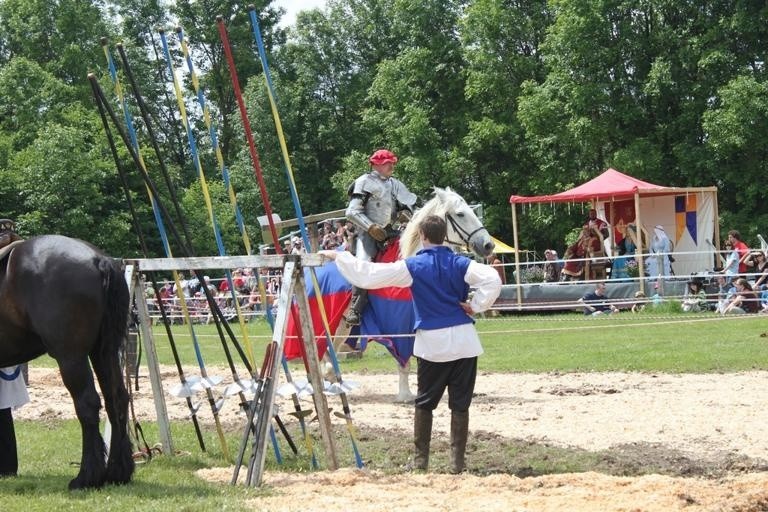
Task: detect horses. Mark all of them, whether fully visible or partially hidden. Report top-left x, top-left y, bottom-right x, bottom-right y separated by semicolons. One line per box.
0;233;136;493
298;185;498;405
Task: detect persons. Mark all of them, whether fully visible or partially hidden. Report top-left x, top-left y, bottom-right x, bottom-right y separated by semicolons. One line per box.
0;217;34;476
581;208;610;280
313;210;504;475
643;223;672;276
575;282;619;316
630;290;648;314
561;224;601;282
608;225;637;282
341;147;421;327
482;250;508;286
681;276;706;313
715;230;768;316
137;212;360;328
544;249;561;283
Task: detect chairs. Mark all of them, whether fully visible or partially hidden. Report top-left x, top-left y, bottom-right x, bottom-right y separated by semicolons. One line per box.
565;225;607;280
669;240;675;276
614;221;650;276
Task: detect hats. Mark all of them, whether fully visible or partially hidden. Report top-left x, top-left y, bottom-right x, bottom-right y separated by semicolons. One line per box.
369;150;397;165
687;279;703;286
544;249;557;255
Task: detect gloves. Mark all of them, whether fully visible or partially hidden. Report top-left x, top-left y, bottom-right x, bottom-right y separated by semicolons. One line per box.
368;224;388;242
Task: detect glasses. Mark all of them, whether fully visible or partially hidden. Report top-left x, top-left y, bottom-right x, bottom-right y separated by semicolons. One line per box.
755;254;763;258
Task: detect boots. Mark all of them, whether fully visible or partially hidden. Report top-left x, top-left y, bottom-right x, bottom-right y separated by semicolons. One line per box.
413;407;433;473
448;410;469;474
343;285;367;325
